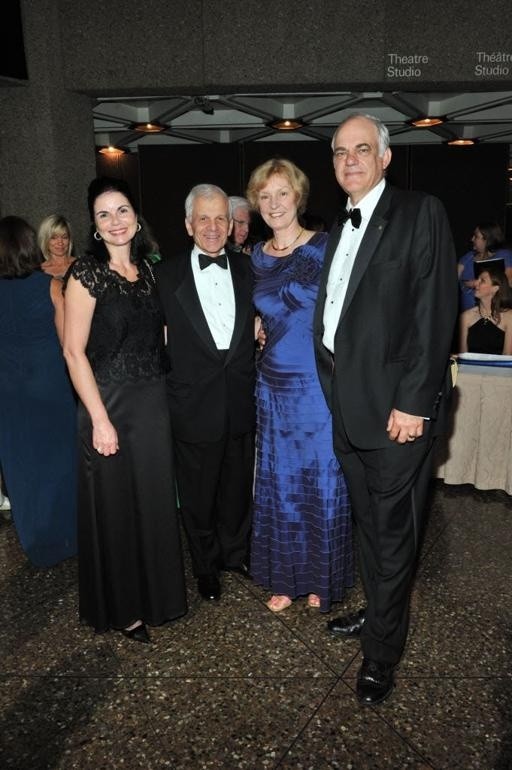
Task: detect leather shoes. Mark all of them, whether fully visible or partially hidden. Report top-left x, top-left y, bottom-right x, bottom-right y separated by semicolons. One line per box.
197;576;223;603
123;621;151;643
234;560;254;580
354;658;396;706
326;609;369;638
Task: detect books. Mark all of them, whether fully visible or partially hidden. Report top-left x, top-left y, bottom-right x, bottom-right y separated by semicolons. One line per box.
473;258;505;279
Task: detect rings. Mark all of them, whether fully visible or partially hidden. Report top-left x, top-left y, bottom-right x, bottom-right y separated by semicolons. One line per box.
96;448;102;452
408;435;415;438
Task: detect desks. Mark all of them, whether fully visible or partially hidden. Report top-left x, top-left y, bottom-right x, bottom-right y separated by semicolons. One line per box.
441;353;512;497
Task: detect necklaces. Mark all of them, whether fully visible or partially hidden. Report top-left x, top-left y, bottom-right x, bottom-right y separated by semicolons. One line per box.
271;228;305;250
479;306;493;324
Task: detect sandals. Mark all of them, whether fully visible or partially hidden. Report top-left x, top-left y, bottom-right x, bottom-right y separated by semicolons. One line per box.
267;595;292;612
309;593;321;607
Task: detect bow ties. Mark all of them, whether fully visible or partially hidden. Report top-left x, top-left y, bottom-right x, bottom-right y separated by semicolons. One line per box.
335;206;360;230
198;252;230;270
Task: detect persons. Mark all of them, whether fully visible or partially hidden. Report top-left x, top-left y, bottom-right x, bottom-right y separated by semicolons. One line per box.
462;270;512;355
155;184;257;603
61;176;186;645
246;157;358;614
39;214;78;279
458;219;511;310
258;113;456;705
227;195;252;255
0;214;82;570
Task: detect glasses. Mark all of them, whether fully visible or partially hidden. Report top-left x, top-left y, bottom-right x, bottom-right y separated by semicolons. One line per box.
233;218;251;227
